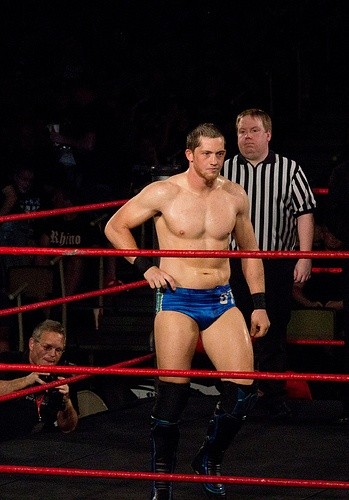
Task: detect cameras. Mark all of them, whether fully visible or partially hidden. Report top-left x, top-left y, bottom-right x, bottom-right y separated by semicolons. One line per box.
31;372;65;419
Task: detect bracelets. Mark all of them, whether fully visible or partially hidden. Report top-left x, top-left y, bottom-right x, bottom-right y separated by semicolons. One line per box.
252;292;266;310
134;257;154;274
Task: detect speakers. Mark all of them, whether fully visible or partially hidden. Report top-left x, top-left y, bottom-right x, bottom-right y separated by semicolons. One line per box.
286;307;338;351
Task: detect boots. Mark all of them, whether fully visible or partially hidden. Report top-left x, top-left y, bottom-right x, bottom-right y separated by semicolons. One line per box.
190;402;247;499
144;412;178;500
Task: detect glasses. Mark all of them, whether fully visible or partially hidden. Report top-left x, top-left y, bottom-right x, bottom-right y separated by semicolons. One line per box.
35;338;64;356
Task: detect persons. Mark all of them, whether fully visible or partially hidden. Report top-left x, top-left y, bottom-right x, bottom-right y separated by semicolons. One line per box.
45;71;117;287
220;109;317;401
0;320;79;441
0;121;90;308
104;122;272;500
